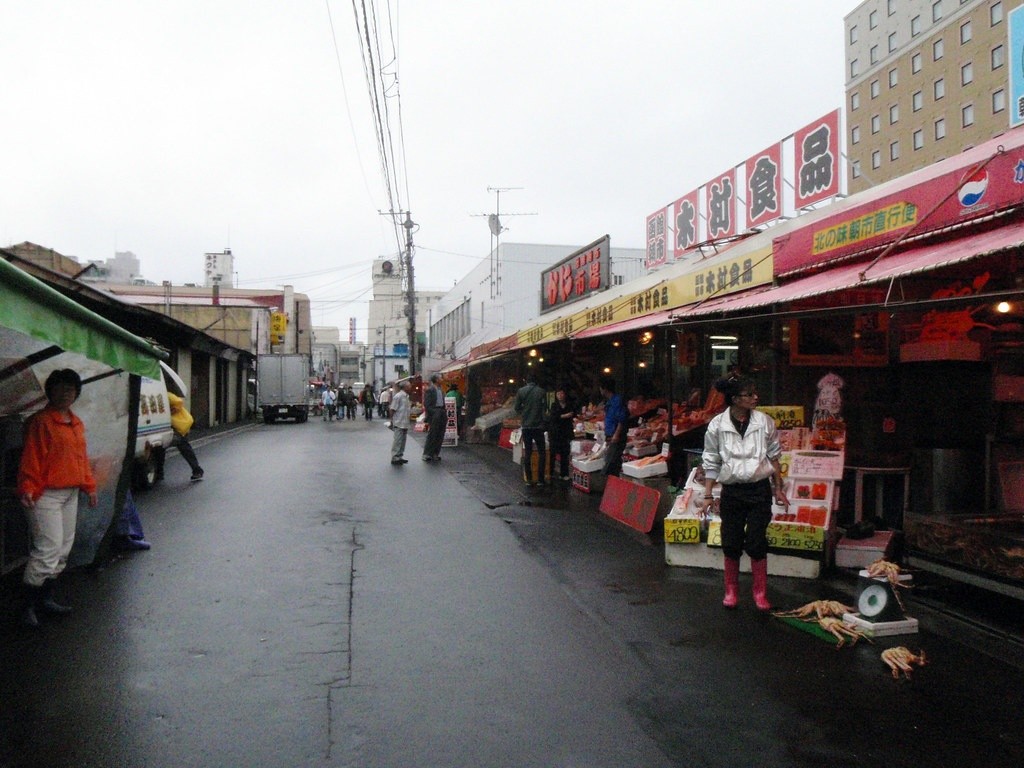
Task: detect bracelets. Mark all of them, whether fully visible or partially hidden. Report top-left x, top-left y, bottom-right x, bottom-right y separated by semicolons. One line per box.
704;495;715;499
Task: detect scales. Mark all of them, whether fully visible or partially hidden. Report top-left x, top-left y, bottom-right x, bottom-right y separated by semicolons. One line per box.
856;571;913;623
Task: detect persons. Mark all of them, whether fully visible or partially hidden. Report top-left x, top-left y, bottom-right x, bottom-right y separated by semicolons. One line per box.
379;387;391;417
514;376;549;486
322;383;356;421
156;391;204;481
548;387;577;483
446;384;464;440
422;372;447;462
361;384;378;421
701;374;791;610
16;368;97;630
599;378;629;476
390;381;411;465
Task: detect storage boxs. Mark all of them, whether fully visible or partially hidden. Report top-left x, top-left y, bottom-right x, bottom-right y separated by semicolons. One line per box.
664;406;896;577
569;438;670;526
995;461;1023;514
511;429;550;481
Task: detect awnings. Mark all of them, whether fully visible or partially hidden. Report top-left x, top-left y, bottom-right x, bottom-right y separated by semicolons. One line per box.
669;216;1024;317
572;285;772;339
438;294;622;377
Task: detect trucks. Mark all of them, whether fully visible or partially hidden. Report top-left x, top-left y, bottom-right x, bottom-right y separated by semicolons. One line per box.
255;351;316;424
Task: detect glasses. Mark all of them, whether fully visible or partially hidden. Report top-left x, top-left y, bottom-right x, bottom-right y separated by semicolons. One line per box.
736;390;760;398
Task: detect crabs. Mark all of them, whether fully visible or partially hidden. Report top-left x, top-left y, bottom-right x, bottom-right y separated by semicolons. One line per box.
819;615;875;651
869;558;922;613
881;645;927;680
774;598;859;622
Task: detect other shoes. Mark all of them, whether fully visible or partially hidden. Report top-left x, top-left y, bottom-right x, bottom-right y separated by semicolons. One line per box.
537;481;546;486
391;458;408;465
525;482;536;486
559;475;570;481
191;473;202;480
423;455;442;461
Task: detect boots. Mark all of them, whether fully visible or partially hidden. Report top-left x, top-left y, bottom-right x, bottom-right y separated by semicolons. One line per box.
723;555;739;607
26;579;75;618
750;557;771;609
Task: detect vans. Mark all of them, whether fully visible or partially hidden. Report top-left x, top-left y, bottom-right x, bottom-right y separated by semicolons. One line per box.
0;351;187;492
352;382;366;399
